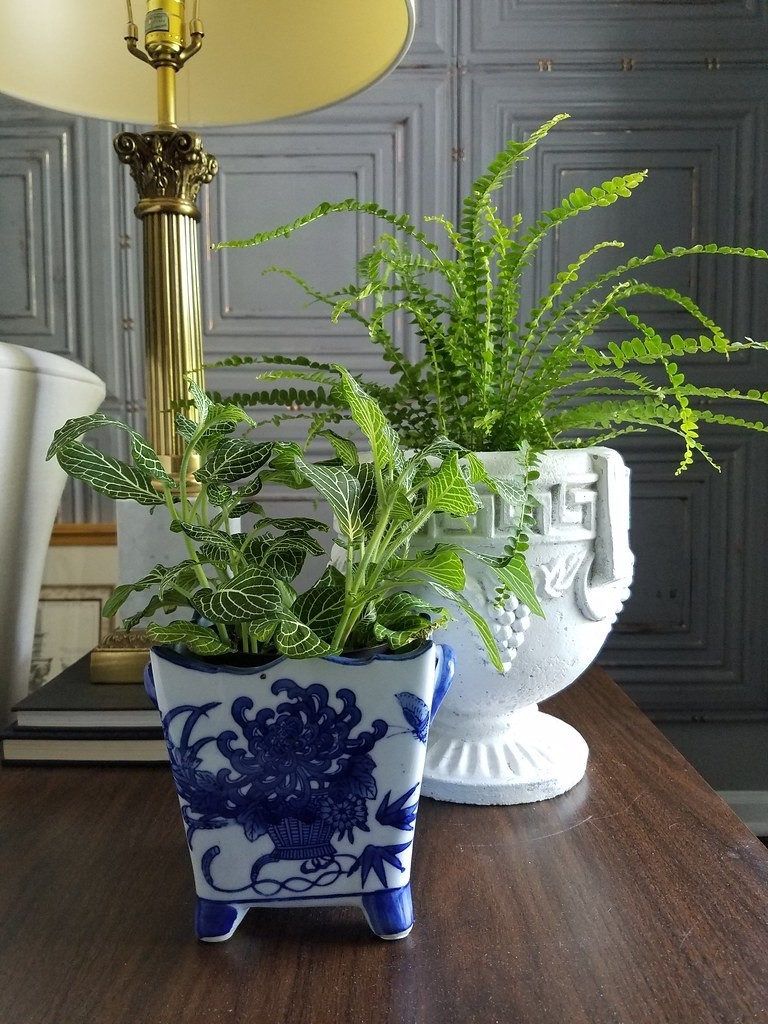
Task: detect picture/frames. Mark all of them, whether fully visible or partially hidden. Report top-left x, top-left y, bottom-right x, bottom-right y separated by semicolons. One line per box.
28;524;120;697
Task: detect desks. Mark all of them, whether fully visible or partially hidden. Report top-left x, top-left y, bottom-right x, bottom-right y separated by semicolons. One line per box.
0;647;768;1024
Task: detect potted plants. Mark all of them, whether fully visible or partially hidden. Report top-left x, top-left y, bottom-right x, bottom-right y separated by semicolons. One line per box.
47;109;768;946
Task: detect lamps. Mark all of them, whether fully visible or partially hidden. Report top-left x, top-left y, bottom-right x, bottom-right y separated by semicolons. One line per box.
0;0;417;686
